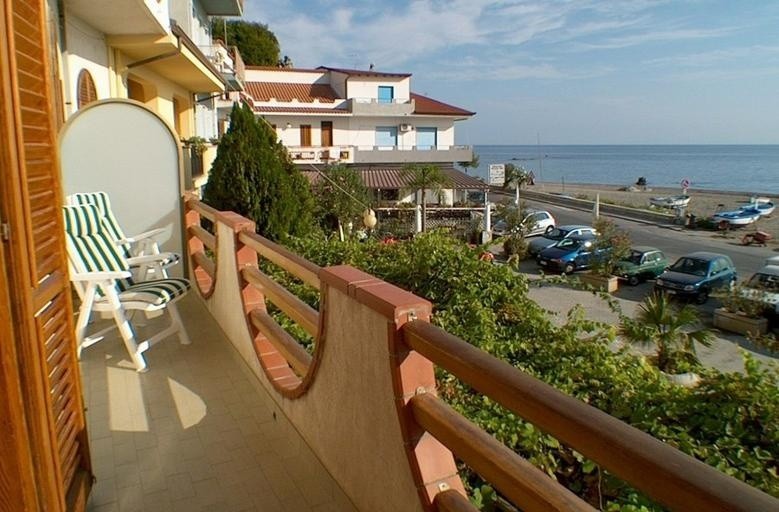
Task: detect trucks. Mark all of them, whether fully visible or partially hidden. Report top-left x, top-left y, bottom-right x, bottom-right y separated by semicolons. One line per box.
739;255;778;326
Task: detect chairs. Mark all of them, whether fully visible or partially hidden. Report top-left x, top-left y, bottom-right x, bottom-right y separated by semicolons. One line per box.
62;202;194;372
65;190;182;321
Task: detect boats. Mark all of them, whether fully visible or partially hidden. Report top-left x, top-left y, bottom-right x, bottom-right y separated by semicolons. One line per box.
711;205;764;228
740;198;775;218
648;193;693;210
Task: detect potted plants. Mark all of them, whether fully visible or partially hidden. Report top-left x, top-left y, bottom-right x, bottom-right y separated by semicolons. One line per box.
580;214;635;295
709;277;778;339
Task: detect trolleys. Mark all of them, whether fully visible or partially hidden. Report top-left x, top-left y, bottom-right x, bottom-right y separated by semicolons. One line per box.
739;223;769;248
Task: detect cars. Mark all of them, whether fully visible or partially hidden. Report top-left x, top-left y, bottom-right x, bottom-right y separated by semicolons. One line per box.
468;191;488;204
652;248;736;304
475;207;664;288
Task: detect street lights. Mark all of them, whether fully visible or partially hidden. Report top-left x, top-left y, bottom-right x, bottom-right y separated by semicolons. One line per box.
363;204;379;242
